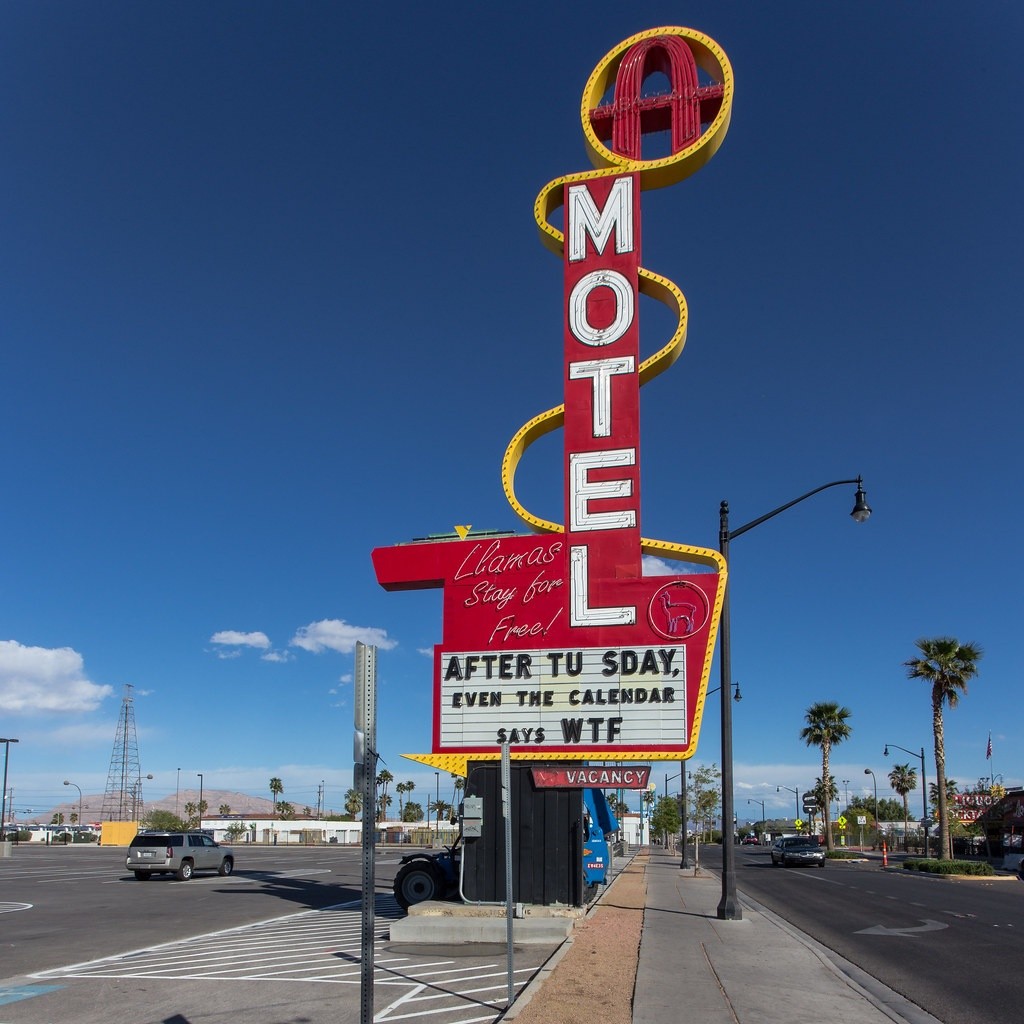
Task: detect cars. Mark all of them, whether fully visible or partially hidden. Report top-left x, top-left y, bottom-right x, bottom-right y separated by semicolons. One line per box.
770;836;826;868
742;834;758;846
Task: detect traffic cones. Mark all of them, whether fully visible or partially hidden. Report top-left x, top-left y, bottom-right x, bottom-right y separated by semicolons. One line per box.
882;841;888;866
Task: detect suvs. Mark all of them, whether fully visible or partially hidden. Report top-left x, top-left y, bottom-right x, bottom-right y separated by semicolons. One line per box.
125;829;235;881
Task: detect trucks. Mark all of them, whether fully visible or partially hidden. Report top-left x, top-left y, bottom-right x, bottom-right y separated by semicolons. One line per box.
392;759;619;913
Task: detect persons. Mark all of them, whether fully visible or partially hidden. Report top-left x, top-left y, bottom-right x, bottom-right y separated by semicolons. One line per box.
652;838;660;845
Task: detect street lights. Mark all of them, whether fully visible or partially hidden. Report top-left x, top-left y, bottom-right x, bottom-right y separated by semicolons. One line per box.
664;770;692;849
72;805;89;833
661;791;678;850
64;781;82;834
681;681;742;871
131;774;153;825
747;799;765;832
1;738;19;841
864;769;876;851
776;785;800;837
883;744;930;857
843;779;850;808
714;474;873;925
